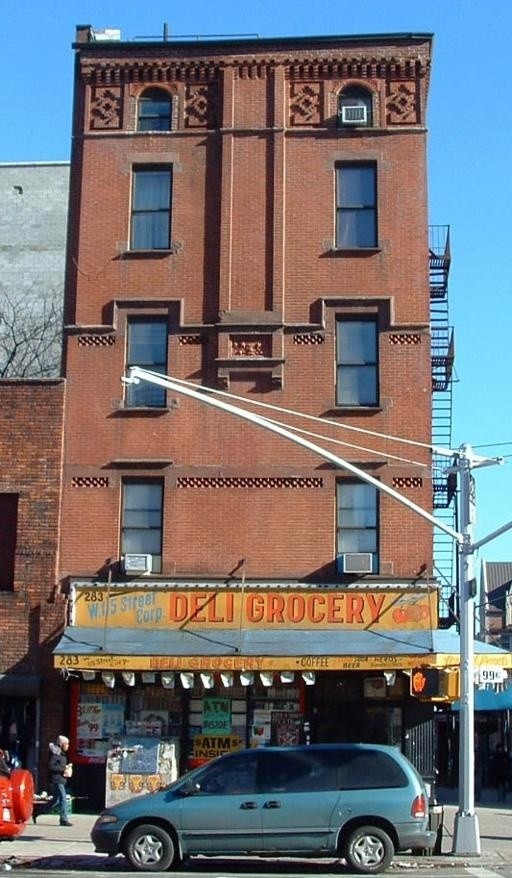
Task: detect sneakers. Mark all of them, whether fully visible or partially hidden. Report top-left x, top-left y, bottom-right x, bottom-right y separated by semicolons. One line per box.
60;820;73;826
33;810;37;823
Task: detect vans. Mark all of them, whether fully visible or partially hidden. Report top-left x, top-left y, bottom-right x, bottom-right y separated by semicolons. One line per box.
92;740;437;874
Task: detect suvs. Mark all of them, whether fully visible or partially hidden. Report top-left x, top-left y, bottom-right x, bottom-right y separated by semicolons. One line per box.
0;751;35;840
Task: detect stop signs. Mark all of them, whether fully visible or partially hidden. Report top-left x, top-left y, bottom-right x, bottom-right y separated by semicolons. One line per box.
411;667;439;697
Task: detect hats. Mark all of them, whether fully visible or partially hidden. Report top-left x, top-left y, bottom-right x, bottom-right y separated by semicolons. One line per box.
57;735;69;745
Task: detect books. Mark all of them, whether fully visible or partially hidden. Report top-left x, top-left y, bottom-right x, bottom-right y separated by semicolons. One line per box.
79;682;299;757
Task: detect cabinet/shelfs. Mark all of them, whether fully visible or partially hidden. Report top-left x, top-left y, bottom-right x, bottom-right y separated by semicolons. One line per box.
75;684;302;770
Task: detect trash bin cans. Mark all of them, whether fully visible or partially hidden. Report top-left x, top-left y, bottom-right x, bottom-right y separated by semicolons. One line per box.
411;802;445;857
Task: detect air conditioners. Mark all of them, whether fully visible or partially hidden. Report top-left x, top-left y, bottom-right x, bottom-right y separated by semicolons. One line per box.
341;105;368;123
124;554;154;575
342;553;373;573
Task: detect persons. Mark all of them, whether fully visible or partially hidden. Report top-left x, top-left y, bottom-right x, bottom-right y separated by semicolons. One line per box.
33;735;74;826
0;750;10;777
493;742;512;801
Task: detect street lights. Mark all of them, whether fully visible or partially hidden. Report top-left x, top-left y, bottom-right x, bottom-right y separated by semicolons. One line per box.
121;365;483;859
